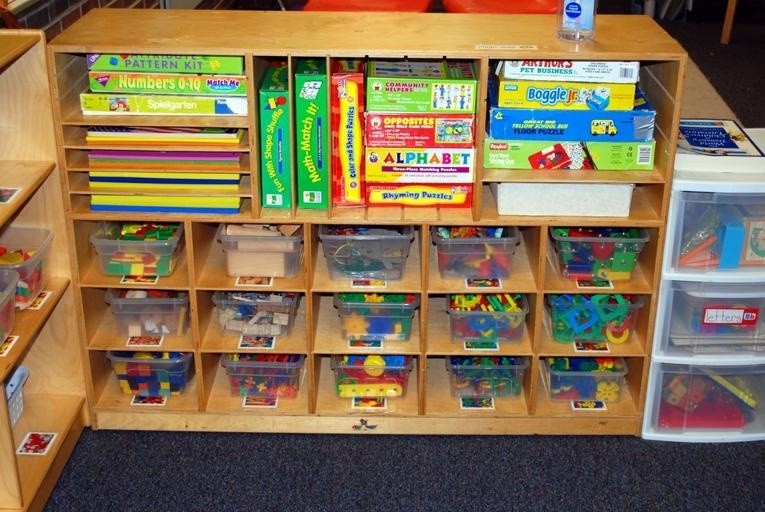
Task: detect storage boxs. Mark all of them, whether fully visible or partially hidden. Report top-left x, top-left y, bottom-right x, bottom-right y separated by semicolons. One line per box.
332;355;413;397
107;353;191;395
670;193;764;276
651;359;763;439
318;226;413;280
333;293;419;339
550;229;649;279
218;225;301;277
446;357;531;397
213;292;299;334
0;267;24;341
220;355;303;396
545;358;629;401
446;296;530;343
106;289;188;336
432;229;520;278
1;225;54;314
90;224;183;275
664;280;765;352
547;297;645;342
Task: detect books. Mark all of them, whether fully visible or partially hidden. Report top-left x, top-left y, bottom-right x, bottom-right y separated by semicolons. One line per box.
673;118;765;174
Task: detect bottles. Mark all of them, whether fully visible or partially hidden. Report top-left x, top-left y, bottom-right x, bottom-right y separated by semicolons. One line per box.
555;1;598;43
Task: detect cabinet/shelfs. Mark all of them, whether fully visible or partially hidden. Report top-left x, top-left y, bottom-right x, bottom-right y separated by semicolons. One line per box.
47;6;690;437
0;28;90;511
636;125;764;446
